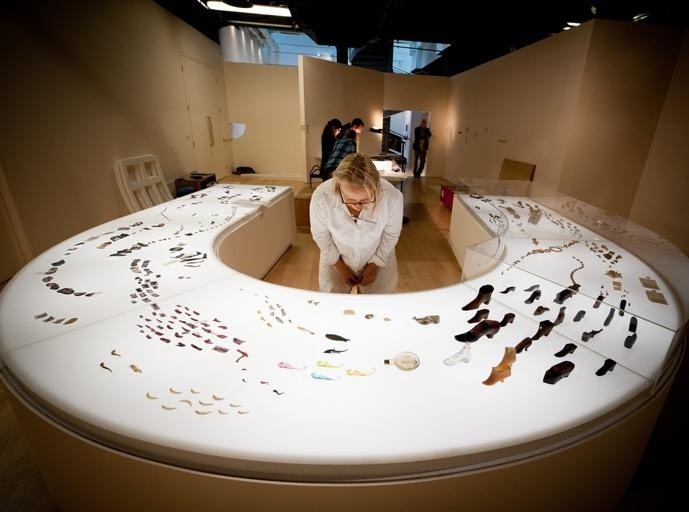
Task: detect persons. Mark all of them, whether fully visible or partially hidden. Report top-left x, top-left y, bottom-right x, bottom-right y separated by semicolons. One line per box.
309;150;404;293
344;117;364;144
320;118;342;182
412;118;431;178
324;129;359;178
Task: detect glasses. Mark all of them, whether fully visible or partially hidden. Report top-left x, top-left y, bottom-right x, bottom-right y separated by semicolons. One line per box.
338;186;377;205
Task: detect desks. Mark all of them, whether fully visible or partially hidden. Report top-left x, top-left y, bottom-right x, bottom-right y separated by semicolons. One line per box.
372;160;410;226
